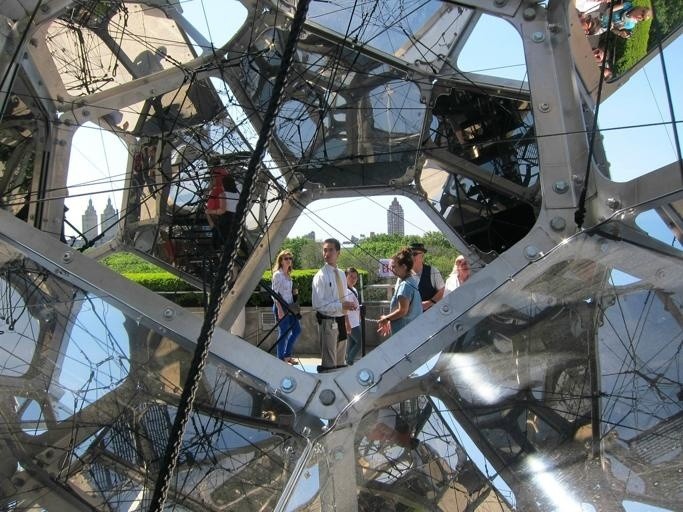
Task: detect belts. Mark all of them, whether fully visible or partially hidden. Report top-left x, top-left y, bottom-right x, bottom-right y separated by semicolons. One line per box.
321;315;335;319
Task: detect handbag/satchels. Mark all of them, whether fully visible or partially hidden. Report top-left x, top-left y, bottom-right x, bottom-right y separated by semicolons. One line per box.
360;305;366;322
287;295;301;316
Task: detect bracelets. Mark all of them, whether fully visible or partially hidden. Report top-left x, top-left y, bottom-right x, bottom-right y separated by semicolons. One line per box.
430;298;436;304
386;315;390;322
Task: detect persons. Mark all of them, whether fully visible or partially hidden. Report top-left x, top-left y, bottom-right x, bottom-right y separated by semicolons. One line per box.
375;247;424;339
341;267;363;364
204;176;241;242
407;242;445;312
270;248;300;366
442;254;470;300
591;45;606;65
311;237;358;370
592;1;652;38
576;0;610;22
599;63;612;82
203;167;228;244
576;8;602;40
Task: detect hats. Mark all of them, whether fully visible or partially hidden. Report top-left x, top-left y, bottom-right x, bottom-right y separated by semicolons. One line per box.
411;243;427;253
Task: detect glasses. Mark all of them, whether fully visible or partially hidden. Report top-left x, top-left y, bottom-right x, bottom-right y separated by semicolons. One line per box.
284;257;292;260
413;252;422;256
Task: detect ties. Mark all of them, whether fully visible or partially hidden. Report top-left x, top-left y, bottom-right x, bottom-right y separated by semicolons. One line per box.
334;269;348;314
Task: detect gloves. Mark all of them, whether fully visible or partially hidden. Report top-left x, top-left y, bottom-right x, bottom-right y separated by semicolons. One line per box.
377;315;391;337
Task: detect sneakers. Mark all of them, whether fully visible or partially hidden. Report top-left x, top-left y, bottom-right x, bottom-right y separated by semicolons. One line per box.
284;358;298;366
